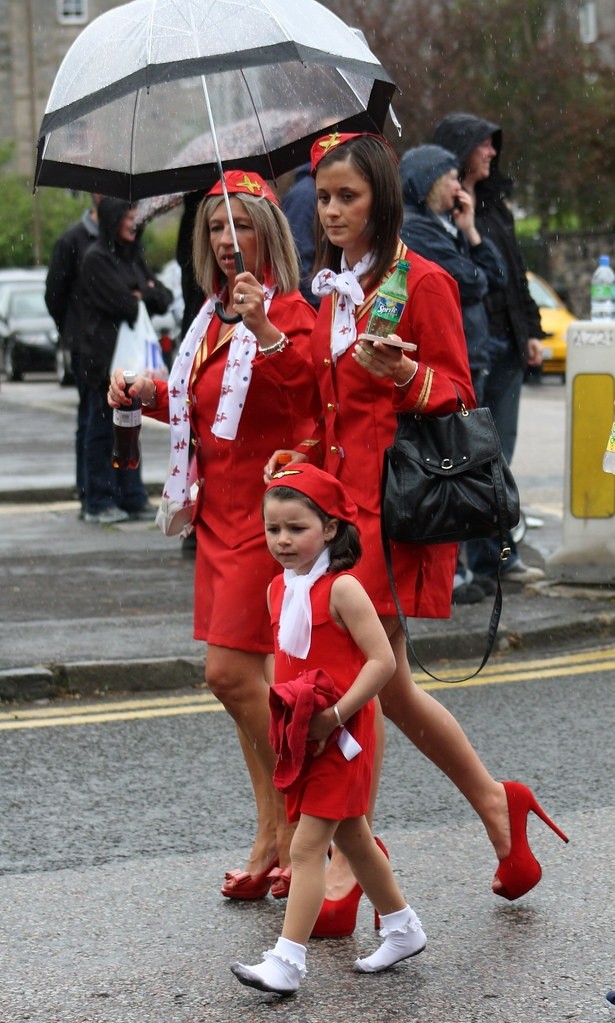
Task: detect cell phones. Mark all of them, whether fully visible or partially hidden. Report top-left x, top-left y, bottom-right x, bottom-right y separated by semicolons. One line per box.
358;334;417;351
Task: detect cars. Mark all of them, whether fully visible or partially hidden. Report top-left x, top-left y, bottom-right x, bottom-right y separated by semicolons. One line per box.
524;269;582;384
0;259;186;391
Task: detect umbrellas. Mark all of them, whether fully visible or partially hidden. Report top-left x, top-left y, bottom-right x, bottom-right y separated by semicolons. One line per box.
32;0;402;324
130;108;317;233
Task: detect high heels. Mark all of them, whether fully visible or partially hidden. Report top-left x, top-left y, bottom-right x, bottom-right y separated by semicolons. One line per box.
220;854;278;900
311;836;388;938
493;781;569;901
269;845;334;897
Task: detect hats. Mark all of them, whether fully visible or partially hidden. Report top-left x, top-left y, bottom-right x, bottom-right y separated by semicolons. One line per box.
206;170;280;207
266;464;358;524
310;133;400;179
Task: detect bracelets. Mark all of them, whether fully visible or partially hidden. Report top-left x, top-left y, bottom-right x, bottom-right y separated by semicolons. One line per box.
333;704;344;728
142;387;156;406
258;332;289;356
394;361;418;387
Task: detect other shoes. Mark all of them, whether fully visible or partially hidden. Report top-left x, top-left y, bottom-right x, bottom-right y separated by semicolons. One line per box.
451;575;485;604
84;504;127;523
473;574;498;597
129;502;158;520
500;561;546;583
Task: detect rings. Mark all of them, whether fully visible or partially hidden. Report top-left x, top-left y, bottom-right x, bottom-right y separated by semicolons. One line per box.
239;293;244;303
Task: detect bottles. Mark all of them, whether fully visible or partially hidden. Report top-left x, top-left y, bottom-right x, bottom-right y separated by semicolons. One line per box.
359;259;411;359
111;370;142;468
590;256;615;321
602;421;615;477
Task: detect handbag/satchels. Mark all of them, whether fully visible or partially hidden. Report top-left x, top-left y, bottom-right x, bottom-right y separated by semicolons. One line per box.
380;387;521;543
110;300;170;384
54;337;77;385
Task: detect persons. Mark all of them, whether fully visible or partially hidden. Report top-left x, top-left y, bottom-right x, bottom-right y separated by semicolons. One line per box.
264;131;569;939
434;113;545;583
231;462;427;995
46;193;158;522
400;144;507;604
179;116;342;557
109;170;333;899
